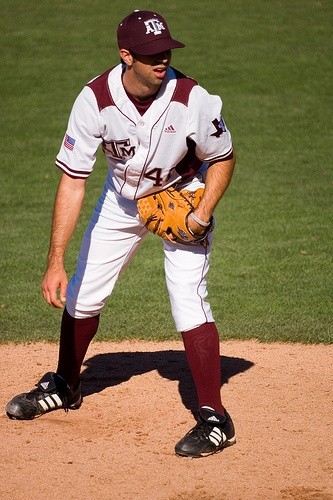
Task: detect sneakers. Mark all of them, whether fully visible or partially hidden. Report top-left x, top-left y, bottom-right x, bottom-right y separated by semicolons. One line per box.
175;405;236;457
4;370;83;419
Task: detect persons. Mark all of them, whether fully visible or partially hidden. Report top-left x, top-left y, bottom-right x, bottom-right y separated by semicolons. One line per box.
5;11;235;457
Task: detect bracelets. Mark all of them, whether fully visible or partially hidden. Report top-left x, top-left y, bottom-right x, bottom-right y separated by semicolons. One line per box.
189;213;211;231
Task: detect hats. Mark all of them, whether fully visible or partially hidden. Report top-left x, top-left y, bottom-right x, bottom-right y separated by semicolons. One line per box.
117;9;185;56
